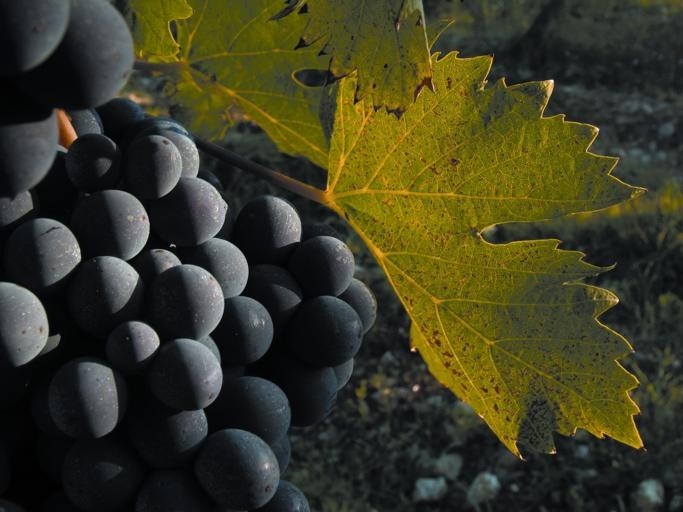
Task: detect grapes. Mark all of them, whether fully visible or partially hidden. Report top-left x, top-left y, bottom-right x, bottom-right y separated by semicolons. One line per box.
0;0;377;512
